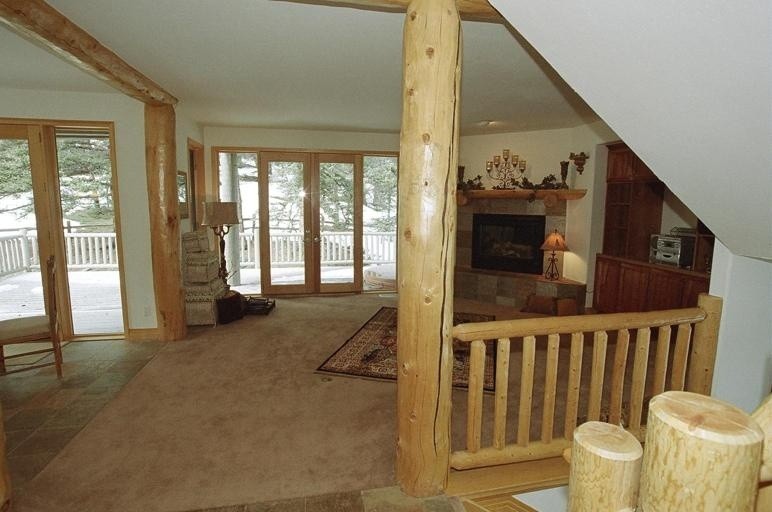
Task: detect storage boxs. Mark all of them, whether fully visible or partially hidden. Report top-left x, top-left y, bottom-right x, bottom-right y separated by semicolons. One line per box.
183;229;222;327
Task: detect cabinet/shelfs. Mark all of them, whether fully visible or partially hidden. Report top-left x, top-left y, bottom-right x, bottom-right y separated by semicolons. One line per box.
602;145;663;261
647;266;710;340
591;254;648;315
692;216;715;274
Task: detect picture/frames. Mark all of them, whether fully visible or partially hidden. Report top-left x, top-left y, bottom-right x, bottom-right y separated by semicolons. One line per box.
176;169;190;219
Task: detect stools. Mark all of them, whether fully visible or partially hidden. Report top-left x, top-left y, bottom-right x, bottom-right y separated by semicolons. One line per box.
644;380;761;512
564;415;643;512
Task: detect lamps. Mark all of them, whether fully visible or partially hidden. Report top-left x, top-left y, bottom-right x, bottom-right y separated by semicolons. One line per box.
543;228;569;278
485;149;528;190
202;203;248;326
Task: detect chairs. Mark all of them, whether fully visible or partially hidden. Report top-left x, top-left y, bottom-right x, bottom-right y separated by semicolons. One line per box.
0;249;65;380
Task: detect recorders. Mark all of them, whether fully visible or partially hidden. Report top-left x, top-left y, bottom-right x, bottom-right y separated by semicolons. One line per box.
648;233;693;268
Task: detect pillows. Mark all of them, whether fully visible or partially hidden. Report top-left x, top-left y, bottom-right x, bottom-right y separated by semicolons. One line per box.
552;293;581;320
516;285;558;317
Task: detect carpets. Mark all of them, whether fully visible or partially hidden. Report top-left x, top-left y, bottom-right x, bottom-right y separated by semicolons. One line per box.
313;306;496;396
